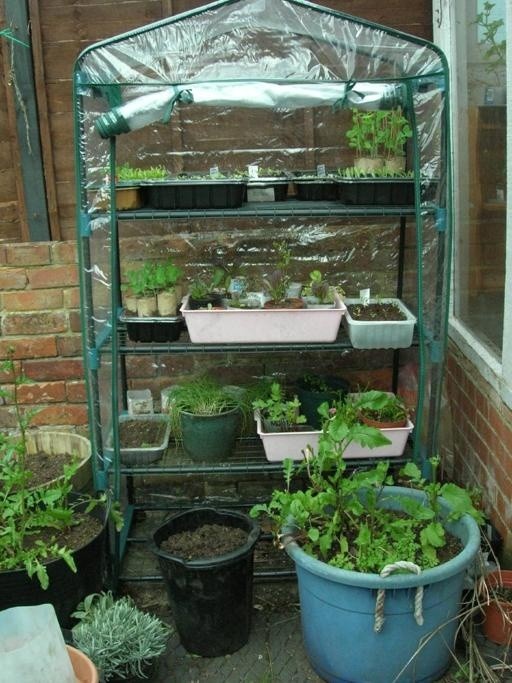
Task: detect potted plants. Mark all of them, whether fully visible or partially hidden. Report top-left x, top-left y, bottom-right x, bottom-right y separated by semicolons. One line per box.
0;430;512;683
87;107;442;430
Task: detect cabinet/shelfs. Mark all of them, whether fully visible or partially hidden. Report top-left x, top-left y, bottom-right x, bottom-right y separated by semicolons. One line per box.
70;0;455;600
